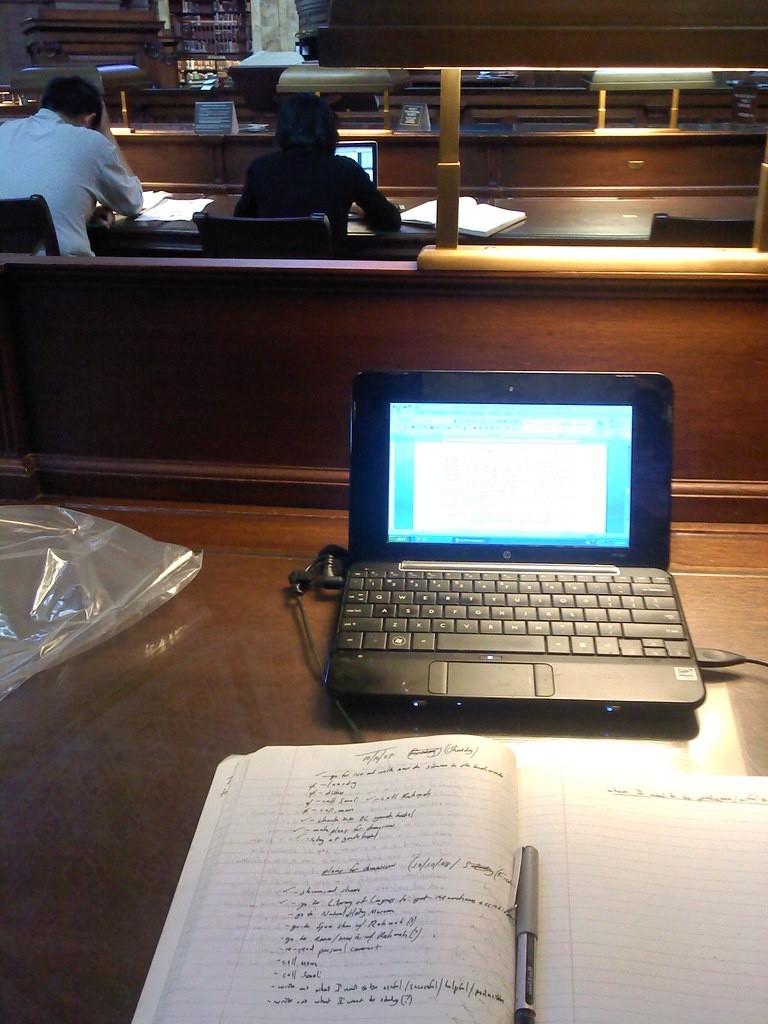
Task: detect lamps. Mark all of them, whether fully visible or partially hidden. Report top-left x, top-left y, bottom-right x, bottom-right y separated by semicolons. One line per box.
329;0;768;277
10;64;145;133
0;85;13;103
589;70;715;136
273;67;414;135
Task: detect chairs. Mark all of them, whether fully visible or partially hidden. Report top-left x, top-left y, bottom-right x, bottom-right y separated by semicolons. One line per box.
0;195;60;257
192;211;341;262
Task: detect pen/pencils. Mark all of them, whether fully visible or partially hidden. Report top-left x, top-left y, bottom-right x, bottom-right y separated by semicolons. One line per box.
505;844;541;1024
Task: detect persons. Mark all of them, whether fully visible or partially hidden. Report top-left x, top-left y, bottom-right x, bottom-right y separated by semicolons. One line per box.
232;92;401;260
1;75;143;258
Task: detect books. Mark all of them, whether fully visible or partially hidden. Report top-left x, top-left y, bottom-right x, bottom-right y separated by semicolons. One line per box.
399;196;527;238
130;734;768;1024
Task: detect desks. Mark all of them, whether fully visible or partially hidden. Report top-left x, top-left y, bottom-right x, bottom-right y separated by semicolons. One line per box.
89;183;764;260
0;450;768;1024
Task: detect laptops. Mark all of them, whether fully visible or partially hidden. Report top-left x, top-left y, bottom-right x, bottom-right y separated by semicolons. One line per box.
330;369;708;710
335;141;378;219
